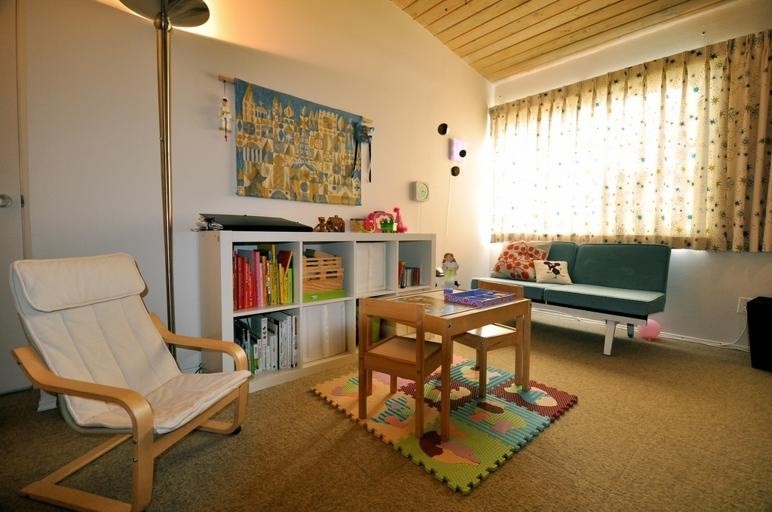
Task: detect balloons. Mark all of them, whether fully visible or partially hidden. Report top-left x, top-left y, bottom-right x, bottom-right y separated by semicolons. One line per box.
638;318;661;341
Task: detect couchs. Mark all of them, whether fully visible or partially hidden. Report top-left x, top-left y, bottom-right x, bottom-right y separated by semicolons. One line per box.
470;240;670;357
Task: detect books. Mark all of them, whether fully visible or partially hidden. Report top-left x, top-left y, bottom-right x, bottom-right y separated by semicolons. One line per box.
398;260;421;289
232;242;299;376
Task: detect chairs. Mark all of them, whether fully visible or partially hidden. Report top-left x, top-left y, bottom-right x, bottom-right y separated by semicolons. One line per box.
8;252;249;512
358;276;532;441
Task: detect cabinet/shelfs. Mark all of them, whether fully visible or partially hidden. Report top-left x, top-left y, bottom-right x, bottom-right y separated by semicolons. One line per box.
197;231;437;392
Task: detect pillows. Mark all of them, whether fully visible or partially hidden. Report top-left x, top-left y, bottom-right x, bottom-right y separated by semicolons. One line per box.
490;241;573;284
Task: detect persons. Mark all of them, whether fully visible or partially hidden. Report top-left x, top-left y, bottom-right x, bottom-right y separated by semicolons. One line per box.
440;253;460;288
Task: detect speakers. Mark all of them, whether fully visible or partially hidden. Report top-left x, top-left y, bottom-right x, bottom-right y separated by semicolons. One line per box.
449;137;468;162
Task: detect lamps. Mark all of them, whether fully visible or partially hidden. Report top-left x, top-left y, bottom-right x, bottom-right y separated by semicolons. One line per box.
118;0;210;372
